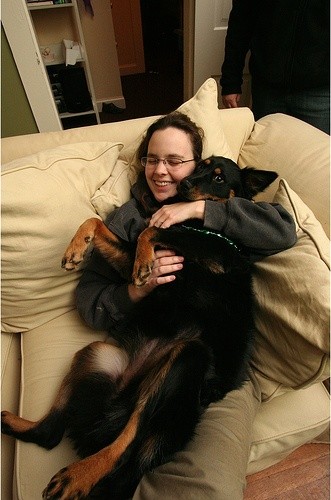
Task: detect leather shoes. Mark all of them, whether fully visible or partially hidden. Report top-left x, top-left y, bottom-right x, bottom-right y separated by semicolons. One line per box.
102;103;123;113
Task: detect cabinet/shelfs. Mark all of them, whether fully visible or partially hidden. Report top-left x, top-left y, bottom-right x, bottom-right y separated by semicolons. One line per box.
23;0;102;129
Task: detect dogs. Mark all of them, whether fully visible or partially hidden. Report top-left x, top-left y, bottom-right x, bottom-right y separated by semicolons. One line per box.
1;155;278;500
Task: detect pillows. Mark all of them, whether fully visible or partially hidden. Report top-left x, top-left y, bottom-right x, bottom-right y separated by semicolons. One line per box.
248;181;330;406
1;141;126;336
90;78;235;221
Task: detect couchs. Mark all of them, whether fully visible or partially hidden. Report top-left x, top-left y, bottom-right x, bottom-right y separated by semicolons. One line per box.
1;106;331;500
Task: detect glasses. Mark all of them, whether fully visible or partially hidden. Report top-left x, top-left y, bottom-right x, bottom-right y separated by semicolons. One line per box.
141;157;195;169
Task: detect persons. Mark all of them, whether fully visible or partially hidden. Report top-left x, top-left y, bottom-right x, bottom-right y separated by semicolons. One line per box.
220;0;330;135
76;112;297;500
